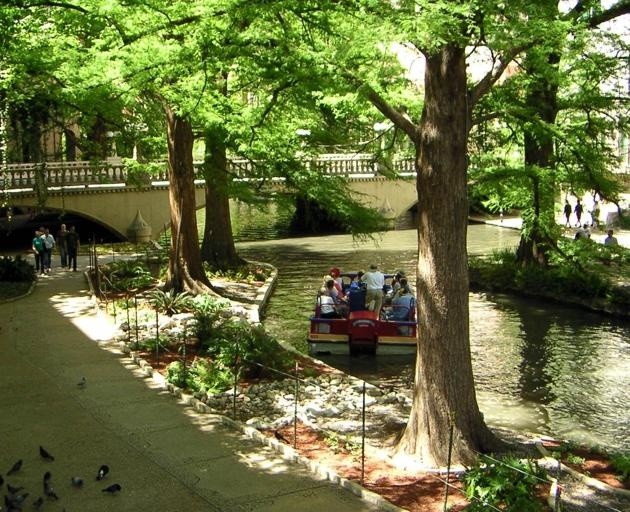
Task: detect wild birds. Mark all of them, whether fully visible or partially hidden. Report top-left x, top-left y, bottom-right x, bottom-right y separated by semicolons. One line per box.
76;376;87;386
101;483;122;495
96;464;109;481
0;459;59;512
70;477;85;488
39;445;55;460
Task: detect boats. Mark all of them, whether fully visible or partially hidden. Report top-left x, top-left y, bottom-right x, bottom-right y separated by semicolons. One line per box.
307;267;418;358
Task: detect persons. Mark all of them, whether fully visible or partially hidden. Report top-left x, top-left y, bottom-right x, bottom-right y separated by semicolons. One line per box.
605;230;617;245
577;224;590;239
562;200;572;228
574;200;583;227
317;263;415;320
591;201;600;226
32;224;80;275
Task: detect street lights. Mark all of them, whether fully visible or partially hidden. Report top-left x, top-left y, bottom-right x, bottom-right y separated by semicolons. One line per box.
373;122;393;156
295;127;312;146
